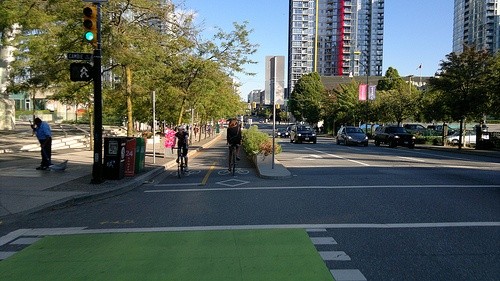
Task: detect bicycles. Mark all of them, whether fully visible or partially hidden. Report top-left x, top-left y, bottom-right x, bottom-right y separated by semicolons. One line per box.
227;142;242;176
171;145;187;178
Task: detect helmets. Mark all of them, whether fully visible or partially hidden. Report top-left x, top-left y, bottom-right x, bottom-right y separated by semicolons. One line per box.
178;124;186;131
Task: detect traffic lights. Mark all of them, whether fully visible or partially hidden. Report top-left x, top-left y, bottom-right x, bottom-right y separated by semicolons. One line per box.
82;6;97;42
70;63;93;82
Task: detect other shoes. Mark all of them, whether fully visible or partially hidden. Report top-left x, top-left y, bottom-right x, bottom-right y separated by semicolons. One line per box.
36;163;52;170
186;167;188;172
236;156;241;160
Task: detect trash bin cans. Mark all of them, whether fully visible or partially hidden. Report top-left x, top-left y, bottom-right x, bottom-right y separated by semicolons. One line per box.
216;124;219;133
124;137;136;176
136;137;145;173
103;136;128;180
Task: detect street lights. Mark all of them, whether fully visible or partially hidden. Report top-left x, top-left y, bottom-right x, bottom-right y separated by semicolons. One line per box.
340;58;368;134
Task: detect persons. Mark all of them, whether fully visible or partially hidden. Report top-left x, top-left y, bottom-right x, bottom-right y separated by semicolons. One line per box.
226;119;241;171
193;125;199;140
30;116;53;171
172;128;190;173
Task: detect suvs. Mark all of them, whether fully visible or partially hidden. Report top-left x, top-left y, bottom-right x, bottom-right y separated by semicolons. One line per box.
373;124;416;149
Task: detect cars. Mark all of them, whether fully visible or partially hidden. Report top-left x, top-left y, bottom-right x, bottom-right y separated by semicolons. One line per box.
290;125;317;144
360;122;458;138
272;125;292;138
335;126;369;146
